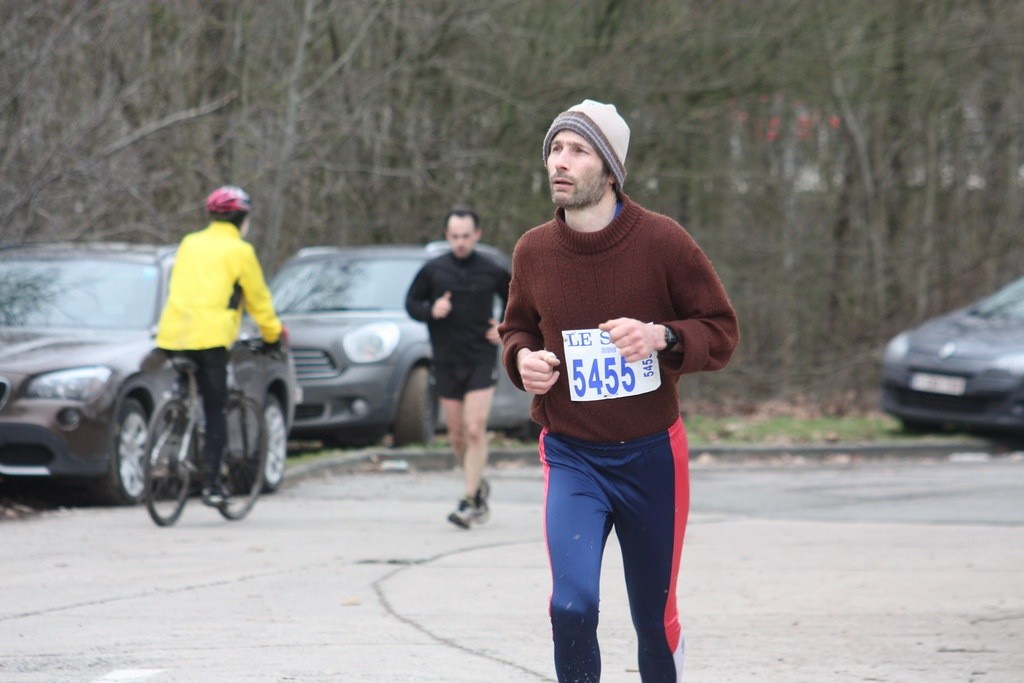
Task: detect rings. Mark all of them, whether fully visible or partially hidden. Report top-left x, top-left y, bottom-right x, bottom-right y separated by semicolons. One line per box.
544;352;557;363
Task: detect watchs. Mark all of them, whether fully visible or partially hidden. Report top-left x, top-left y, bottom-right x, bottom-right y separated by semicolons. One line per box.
658;327;677;356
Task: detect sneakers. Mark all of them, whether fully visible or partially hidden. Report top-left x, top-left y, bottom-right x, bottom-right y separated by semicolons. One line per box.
476;480;491;500
447;496;490;529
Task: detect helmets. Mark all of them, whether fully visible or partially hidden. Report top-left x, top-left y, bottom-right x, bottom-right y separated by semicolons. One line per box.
205;184;252;217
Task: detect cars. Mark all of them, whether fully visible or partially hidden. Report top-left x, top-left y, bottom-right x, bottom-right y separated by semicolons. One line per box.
873;273;1024;444
0;240;306;503
240;238;539;445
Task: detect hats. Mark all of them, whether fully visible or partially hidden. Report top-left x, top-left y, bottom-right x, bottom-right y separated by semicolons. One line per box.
542;98;631;194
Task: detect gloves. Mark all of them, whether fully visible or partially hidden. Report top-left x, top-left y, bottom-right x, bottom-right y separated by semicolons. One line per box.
281;323;289;345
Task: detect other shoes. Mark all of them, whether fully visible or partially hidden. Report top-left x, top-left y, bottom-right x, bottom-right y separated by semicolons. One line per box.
203;487;232;504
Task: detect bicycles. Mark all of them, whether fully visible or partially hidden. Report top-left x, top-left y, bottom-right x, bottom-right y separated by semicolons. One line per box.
141;332;287;528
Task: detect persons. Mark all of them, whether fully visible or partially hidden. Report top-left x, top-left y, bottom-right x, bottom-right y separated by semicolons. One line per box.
406;206;511;528
497;98;739;682
155;184;288;508
